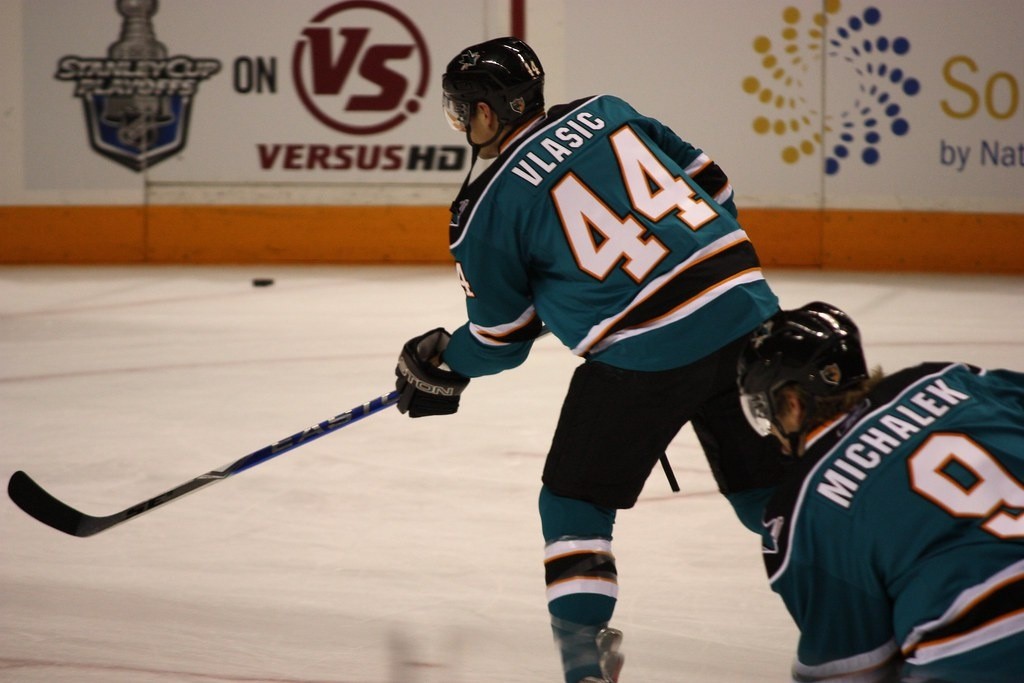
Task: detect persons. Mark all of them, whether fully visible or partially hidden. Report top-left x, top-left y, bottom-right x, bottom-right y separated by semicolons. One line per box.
395;35;790;683
736;300;1024;683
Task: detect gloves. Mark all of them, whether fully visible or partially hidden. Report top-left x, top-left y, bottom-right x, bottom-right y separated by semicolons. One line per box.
394;325;471;419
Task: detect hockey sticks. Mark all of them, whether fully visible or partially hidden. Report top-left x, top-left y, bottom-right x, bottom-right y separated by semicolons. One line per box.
7;390;402;538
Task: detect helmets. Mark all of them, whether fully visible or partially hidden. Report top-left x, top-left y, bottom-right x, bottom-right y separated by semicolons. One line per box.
757;301;869;395
442;36;545;122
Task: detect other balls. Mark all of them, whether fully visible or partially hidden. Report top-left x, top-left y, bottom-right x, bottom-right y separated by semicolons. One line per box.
254;278;274;286
